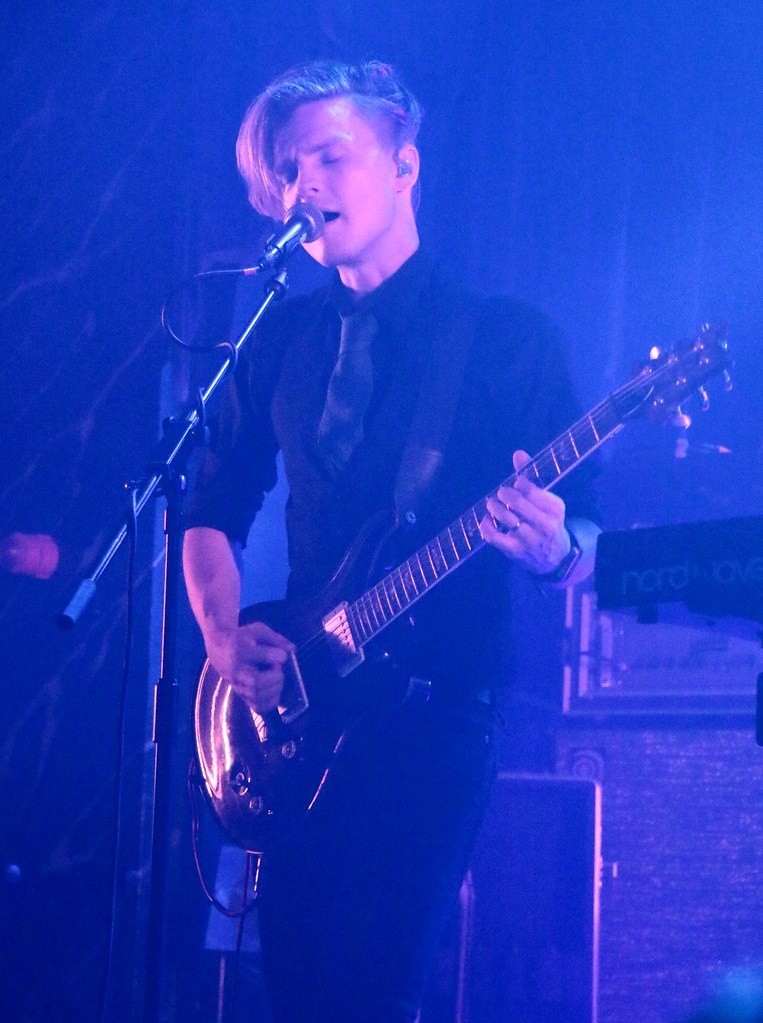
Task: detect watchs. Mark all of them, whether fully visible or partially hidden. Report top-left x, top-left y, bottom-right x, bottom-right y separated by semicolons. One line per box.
534;522;584;586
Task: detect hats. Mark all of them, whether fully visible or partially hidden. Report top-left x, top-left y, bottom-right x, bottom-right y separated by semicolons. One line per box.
3;505;61;536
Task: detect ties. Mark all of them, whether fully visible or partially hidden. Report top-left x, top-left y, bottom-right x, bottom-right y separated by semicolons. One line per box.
318;309;380;473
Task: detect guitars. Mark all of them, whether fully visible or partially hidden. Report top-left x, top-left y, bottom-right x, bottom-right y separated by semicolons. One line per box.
195;322;737;853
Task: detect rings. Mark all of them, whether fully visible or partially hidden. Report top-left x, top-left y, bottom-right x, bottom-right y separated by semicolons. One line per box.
510;517;525;533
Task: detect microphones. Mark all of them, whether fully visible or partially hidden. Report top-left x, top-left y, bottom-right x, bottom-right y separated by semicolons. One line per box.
257;205;326;271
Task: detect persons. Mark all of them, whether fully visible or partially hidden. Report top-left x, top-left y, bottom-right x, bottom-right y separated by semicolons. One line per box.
165;48;630;1023
